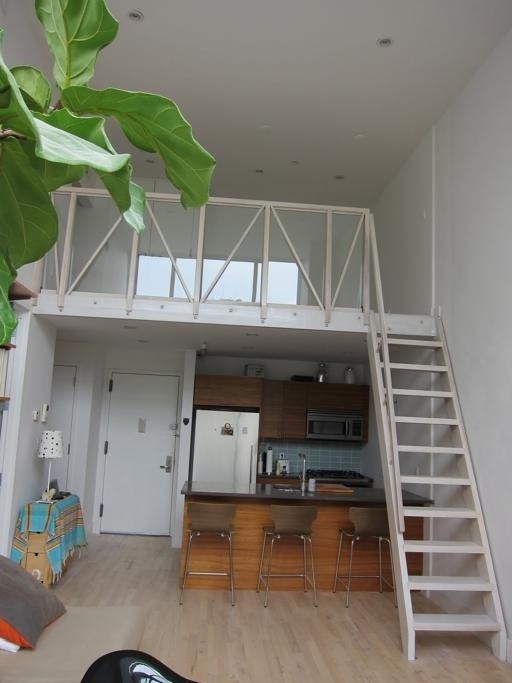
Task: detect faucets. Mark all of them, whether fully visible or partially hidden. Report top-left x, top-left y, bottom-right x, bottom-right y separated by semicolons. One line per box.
298;452;307;490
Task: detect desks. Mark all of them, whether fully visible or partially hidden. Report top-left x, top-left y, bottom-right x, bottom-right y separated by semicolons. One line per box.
10;495;87;587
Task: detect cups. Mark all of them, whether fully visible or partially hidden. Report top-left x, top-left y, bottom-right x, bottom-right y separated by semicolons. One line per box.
307;478;316;492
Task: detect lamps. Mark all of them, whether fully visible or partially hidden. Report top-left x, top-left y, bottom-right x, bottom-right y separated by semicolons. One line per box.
36;431;63;504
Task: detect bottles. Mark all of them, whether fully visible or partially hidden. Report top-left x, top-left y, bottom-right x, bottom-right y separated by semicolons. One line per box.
281;466;286;474
266;446;272;473
317;362;327;383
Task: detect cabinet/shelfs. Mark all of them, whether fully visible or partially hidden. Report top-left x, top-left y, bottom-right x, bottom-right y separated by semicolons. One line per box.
194;375;369;442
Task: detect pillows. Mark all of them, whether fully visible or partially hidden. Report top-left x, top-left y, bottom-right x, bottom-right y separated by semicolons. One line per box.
0;554;67;649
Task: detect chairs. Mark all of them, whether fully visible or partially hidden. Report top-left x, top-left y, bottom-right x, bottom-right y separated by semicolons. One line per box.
179;502;237;606
256;505;317;608
333;507;398;608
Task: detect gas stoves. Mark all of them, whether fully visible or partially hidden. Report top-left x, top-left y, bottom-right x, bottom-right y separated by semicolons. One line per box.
308;468;372;487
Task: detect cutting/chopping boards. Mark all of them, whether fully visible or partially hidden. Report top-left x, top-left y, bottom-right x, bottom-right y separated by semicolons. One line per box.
304;483;354;493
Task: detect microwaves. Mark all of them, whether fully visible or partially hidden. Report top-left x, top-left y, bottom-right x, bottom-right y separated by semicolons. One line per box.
306;411;363;444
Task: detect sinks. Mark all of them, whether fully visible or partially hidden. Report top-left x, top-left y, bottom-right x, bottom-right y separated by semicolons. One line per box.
272;484;301;491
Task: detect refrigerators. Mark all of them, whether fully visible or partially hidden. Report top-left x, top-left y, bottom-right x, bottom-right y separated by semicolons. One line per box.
192;410;259;483
